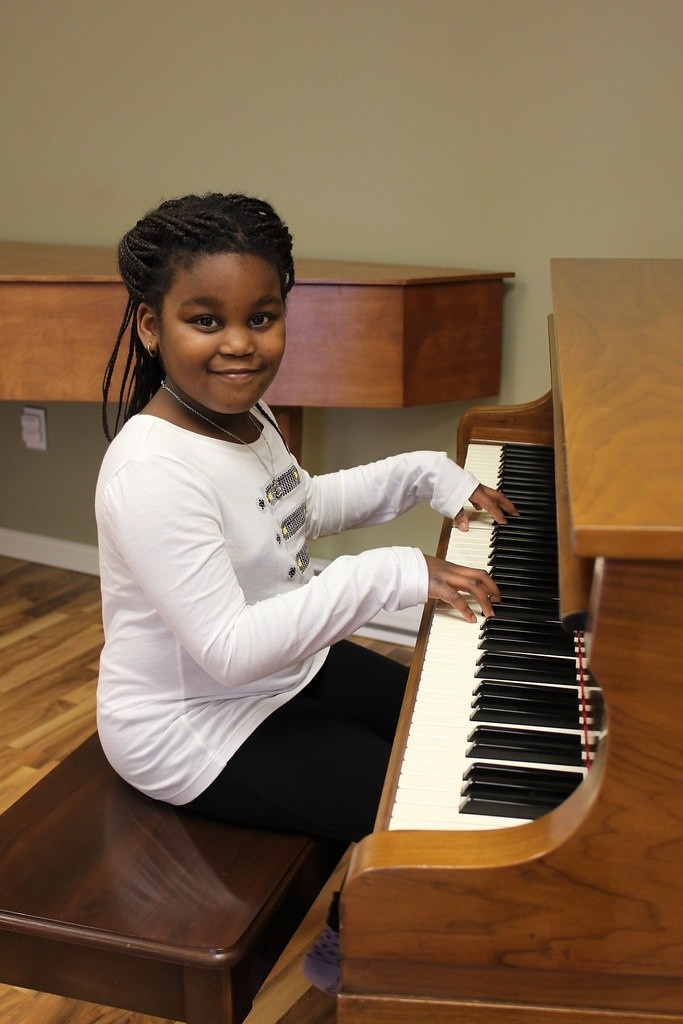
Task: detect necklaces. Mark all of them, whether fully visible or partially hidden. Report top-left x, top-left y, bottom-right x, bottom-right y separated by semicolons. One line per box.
159;378;286;498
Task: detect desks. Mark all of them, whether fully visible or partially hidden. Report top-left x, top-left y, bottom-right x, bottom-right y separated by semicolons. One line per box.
1;242;518;470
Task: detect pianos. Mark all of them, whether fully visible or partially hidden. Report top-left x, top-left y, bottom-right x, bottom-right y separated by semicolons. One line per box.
335;256;683;1024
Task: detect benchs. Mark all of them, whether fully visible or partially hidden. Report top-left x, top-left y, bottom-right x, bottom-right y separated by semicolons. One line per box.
1;714;354;1023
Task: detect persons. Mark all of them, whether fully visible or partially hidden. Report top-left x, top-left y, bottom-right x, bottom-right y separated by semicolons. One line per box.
95;195;520;996
